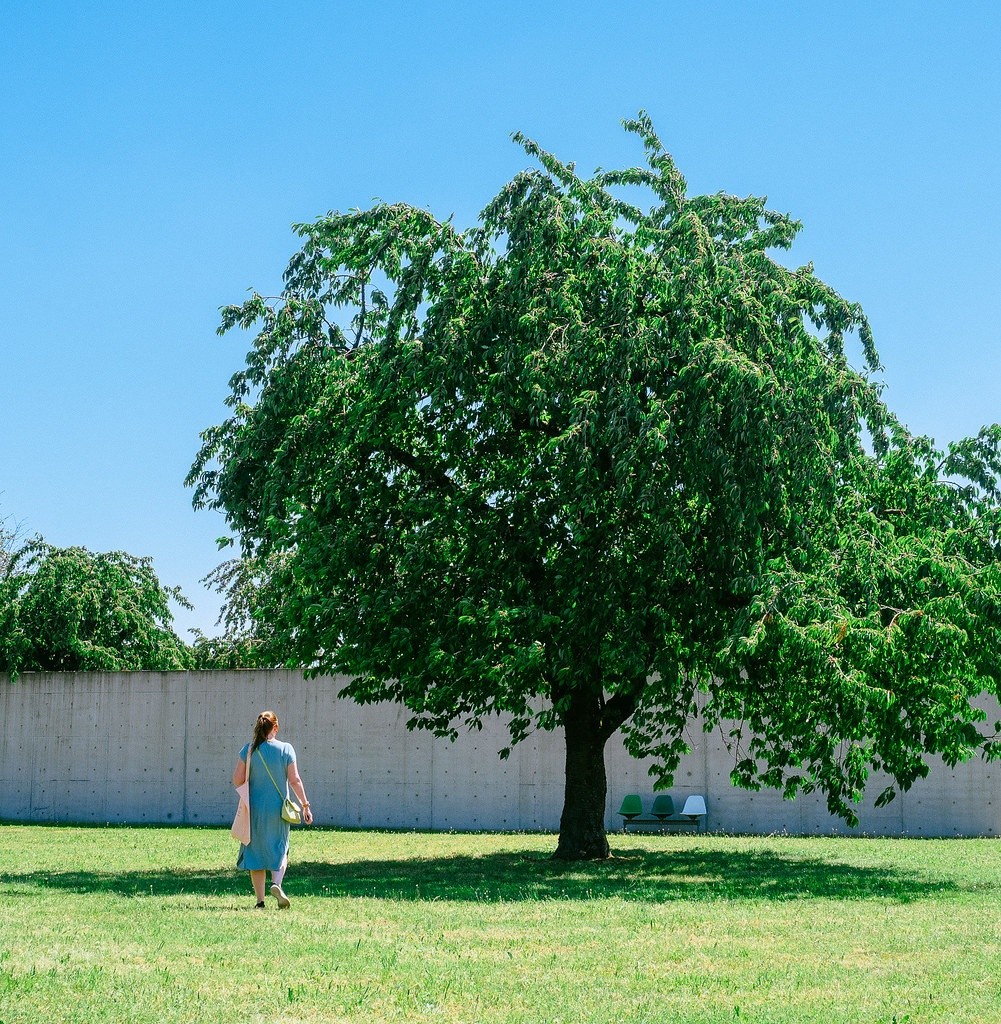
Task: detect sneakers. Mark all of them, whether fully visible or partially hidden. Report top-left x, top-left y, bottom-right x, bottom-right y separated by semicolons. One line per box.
254;901;265;909
270;883;291;909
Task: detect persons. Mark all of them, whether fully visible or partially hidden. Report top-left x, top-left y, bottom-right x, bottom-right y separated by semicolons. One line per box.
233;710;313;910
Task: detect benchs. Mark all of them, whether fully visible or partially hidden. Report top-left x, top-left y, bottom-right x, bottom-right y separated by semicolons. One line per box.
616;795;707;834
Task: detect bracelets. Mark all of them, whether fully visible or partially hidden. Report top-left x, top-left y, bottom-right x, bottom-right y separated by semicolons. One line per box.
302;801;310;808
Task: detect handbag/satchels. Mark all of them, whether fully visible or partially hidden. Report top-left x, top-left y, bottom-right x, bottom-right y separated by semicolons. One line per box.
230;742;251;846
281;797;301;824
